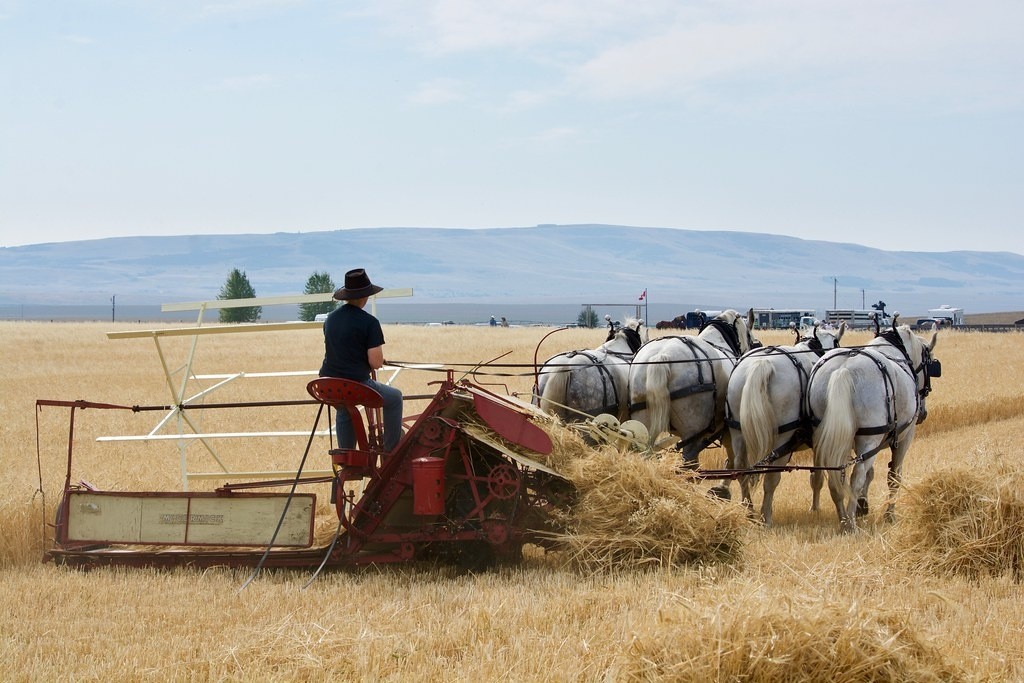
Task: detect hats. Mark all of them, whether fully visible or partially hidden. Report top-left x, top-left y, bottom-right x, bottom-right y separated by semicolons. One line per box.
333;269;384;300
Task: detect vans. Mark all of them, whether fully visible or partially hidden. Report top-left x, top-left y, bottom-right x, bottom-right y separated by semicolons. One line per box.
315;312;328;322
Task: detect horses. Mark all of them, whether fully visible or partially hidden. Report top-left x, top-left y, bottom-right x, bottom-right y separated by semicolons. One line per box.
529;308;937;534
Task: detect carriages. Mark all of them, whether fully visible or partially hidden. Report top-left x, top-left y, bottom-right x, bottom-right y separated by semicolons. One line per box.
32;287;941;579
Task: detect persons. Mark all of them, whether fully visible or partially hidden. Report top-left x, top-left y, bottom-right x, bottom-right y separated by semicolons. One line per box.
318;269;403;458
753;317;796;329
501;317;509;327
490;315;497;326
814;318;849;331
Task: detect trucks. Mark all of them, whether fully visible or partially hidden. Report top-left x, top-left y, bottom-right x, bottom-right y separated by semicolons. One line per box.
927;303;965;326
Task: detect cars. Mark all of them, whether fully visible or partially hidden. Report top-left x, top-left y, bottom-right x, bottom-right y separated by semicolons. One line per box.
442;320;456;326
425;322;443;328
563;321;578;328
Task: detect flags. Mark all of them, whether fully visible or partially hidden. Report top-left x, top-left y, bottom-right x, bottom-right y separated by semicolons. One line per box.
639;289;646;301
